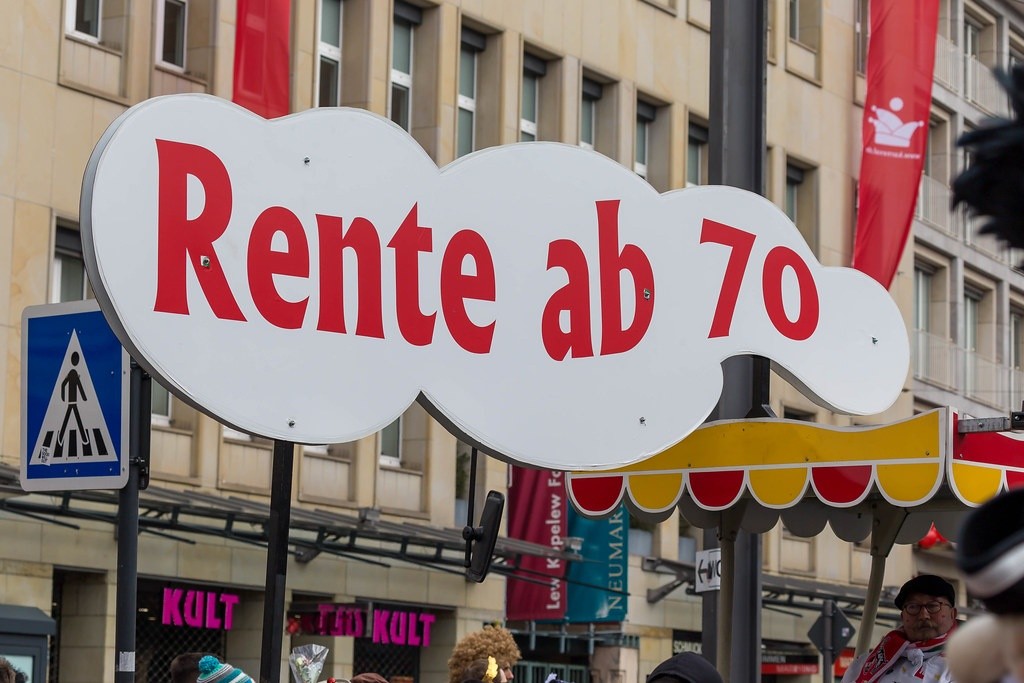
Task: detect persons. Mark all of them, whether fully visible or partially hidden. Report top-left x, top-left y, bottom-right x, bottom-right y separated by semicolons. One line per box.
839;575;958;683
647;651;723;683
446;625;521;683
947;488;1024;683
170;652;256;683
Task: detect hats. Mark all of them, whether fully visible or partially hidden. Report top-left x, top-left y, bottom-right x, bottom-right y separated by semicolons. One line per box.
648;652;723;683
196;655;256;683
895;575;955;611
958;488;1024;598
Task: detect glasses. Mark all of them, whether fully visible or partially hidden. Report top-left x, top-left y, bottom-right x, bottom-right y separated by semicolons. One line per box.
902;600;953;615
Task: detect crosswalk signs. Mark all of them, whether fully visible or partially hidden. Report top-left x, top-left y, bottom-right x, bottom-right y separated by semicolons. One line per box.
20;296;130;490
